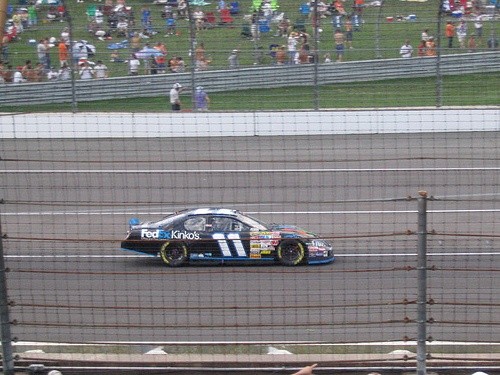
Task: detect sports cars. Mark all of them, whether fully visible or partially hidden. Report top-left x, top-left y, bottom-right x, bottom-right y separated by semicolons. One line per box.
120;207;335;268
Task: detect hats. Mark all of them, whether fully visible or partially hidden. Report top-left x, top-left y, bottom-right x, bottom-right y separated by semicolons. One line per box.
173;83;182;88
196;86;204;90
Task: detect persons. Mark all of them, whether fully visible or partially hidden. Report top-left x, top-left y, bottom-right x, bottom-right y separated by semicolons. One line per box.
0;0;361;84
169;83;183;110
399;0;500;58
193;86;210;111
